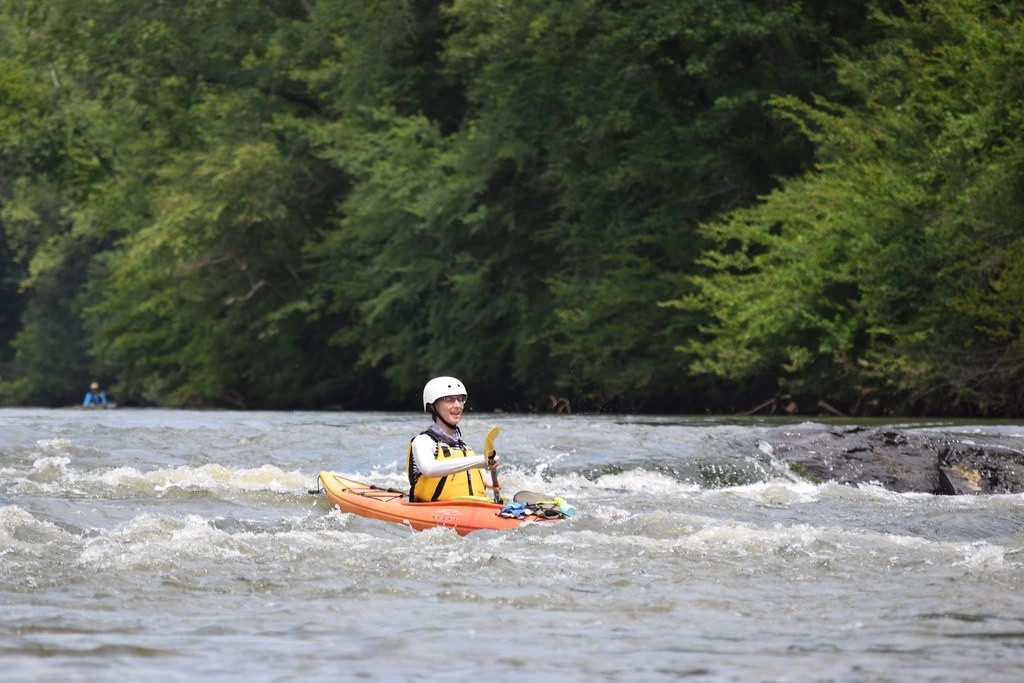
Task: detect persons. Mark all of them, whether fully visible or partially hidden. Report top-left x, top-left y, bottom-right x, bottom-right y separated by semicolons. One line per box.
83;381;108;407
407;376;502;502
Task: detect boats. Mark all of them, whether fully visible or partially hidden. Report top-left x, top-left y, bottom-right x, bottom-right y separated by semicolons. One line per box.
74;403;119;409
308;471;576;543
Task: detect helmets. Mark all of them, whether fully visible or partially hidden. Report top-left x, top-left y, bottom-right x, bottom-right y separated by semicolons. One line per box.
422;376;469;415
89;382;99;389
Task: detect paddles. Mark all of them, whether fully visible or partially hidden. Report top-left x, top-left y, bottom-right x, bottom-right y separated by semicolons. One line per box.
483;426;505;504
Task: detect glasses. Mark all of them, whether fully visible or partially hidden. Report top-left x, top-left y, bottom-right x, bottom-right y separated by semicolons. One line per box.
439;395;467;403
